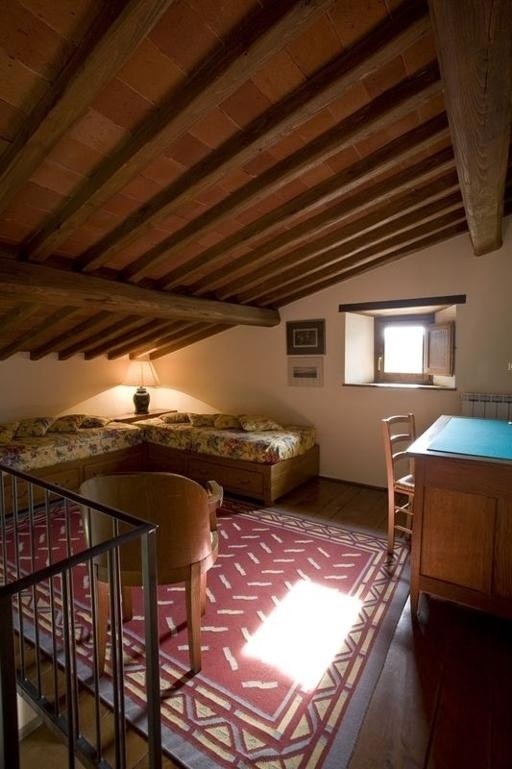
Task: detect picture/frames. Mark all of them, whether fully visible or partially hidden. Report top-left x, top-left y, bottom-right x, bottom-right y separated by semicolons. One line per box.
286;320;324;355
287;357;323;387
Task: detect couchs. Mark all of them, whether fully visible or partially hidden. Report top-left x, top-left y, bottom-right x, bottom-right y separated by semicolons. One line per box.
0;414;319;516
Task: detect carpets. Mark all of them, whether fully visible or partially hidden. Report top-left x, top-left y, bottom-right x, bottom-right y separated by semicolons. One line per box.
0;494;412;769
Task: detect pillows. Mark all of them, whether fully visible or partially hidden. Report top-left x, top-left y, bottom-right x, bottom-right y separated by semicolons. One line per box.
79;416;113;429
48;415;89;433
239;416;284;432
16;417;57;437
188;413;215;427
0;422;20;447
159;413;190;423
213;414;243;429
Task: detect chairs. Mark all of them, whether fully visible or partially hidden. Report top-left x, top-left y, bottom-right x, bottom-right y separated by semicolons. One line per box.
79;470;224;674
381;414;414;555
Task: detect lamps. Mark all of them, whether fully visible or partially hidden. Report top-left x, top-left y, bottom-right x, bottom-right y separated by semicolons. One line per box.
123;359;159;414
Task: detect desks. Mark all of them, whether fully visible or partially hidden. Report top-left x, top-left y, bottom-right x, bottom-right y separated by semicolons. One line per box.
405;415;512;620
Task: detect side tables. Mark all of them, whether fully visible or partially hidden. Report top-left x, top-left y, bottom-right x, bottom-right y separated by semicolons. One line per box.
112;410;177;423
